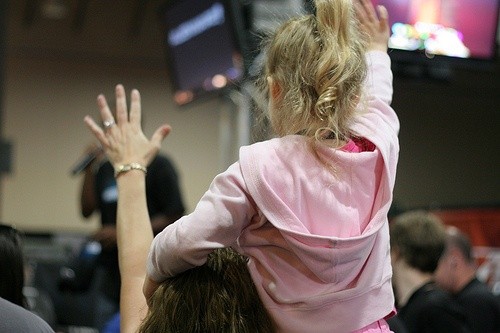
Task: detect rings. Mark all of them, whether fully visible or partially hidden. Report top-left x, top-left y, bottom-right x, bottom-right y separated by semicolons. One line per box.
99;115;120;136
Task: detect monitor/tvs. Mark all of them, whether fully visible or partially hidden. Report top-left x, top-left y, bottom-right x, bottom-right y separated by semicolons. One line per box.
164;0;253;108
372;0;500;82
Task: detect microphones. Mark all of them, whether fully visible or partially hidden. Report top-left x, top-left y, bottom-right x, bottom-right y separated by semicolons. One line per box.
72;154;96;175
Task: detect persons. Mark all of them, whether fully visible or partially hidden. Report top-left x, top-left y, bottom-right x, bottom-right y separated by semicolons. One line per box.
68;137;188;270
141;0;403;333
83;80;283;333
0;225;49;330
391;208;496;333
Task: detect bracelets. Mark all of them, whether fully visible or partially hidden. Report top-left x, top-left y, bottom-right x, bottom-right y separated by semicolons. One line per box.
110;159;149;181
